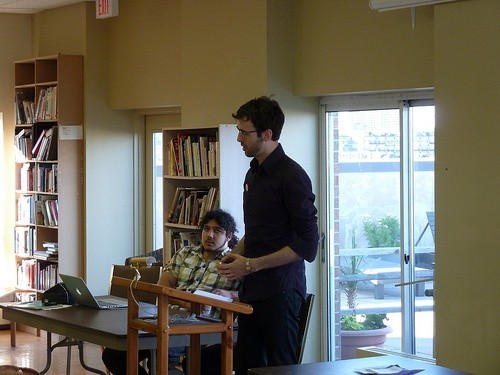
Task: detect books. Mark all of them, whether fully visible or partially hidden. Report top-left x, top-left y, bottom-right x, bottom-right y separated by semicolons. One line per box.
14;86;58;303
166;185;221;226
164;229;202;267
165;134;219;177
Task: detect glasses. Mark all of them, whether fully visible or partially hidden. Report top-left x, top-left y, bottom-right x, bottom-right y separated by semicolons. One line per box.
236;126;257;136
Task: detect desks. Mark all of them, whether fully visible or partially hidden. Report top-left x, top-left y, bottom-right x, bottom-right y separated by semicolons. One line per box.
0;294;239;375
235;354;475;375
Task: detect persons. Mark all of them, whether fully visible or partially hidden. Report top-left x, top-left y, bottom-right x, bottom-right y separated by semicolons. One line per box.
217;94;318;371
101;210;241;375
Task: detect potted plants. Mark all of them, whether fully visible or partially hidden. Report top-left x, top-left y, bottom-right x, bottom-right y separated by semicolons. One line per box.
339;225;394;361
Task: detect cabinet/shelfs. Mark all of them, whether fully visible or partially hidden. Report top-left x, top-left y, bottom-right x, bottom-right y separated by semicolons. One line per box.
12;52;83;341
162;124;255;372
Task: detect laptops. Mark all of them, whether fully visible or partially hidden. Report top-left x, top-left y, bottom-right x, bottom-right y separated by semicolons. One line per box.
59;273;129;309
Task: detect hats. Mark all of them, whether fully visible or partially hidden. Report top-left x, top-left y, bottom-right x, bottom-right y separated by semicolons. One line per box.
42;282;76;305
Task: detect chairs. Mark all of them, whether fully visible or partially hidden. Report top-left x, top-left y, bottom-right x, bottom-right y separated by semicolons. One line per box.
297;291;316;365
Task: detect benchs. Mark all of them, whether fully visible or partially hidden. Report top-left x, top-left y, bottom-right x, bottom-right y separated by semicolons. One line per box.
110;246;164;305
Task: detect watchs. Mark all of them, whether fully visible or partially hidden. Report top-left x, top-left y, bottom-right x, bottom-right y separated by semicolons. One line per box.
245;257;252;274
